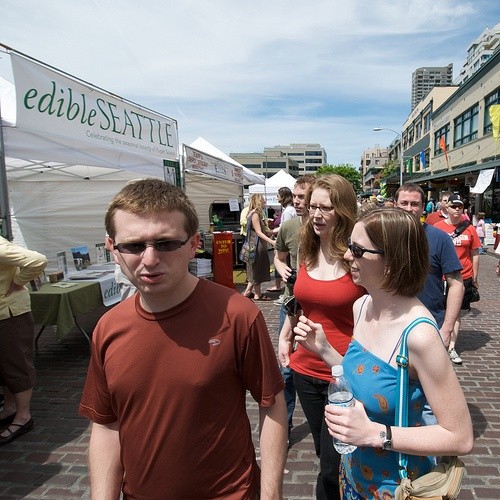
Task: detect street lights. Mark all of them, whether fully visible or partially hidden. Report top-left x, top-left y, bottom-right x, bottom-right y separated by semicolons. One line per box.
373;128;404;187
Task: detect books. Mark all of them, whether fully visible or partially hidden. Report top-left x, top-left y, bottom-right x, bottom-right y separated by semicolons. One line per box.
189;257;212;277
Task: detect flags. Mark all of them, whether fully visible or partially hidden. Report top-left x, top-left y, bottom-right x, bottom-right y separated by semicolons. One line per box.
488;104;500;143
439;135;449;166
419;147;430;170
404;159;413;174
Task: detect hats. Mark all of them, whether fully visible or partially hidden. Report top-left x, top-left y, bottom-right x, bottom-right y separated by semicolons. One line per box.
448;194;464;204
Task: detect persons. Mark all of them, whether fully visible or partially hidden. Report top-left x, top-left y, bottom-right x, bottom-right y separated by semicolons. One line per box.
393;183;466;353
277;171;371;500
299;207;474;500
238;182;486;365
0;233;49;445
105;233;138;300
264;174;316;453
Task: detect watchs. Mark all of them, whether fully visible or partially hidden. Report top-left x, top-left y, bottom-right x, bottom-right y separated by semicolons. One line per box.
382;425;396;455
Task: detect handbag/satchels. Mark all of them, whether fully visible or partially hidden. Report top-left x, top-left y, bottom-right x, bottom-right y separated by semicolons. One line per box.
394;456;465;500
239;242;257;265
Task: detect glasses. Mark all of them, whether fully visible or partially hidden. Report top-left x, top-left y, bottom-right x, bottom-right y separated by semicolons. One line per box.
448;205;463;209
399;201;423;207
305;203;335;214
112;233;192;253
347;238;385;258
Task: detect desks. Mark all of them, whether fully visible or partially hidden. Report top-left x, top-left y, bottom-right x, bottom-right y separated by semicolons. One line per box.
29;281;104;351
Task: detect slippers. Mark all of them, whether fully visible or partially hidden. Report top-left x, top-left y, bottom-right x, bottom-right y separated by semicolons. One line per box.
245;292;272;301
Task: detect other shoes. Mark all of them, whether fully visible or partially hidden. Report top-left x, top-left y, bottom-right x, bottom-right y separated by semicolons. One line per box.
448;346;462;364
0;404;34;445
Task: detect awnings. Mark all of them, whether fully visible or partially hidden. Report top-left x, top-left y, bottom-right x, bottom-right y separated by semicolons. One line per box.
247;168;304;207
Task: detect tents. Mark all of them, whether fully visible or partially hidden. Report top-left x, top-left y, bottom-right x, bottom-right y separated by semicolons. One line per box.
76;178;291;500
0;42;183;289
180;135;266;250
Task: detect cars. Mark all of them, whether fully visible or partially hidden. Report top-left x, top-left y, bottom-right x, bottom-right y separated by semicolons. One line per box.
358;191;382;198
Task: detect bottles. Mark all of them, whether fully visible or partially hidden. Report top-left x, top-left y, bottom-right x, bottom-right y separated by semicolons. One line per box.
328;365;357;455
